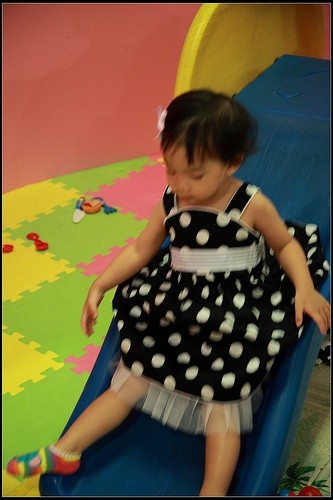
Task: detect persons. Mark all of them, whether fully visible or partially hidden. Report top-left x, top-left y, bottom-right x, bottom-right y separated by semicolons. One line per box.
1;89;333;499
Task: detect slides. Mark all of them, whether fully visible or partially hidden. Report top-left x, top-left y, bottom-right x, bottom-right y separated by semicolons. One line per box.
39;53;332;499
